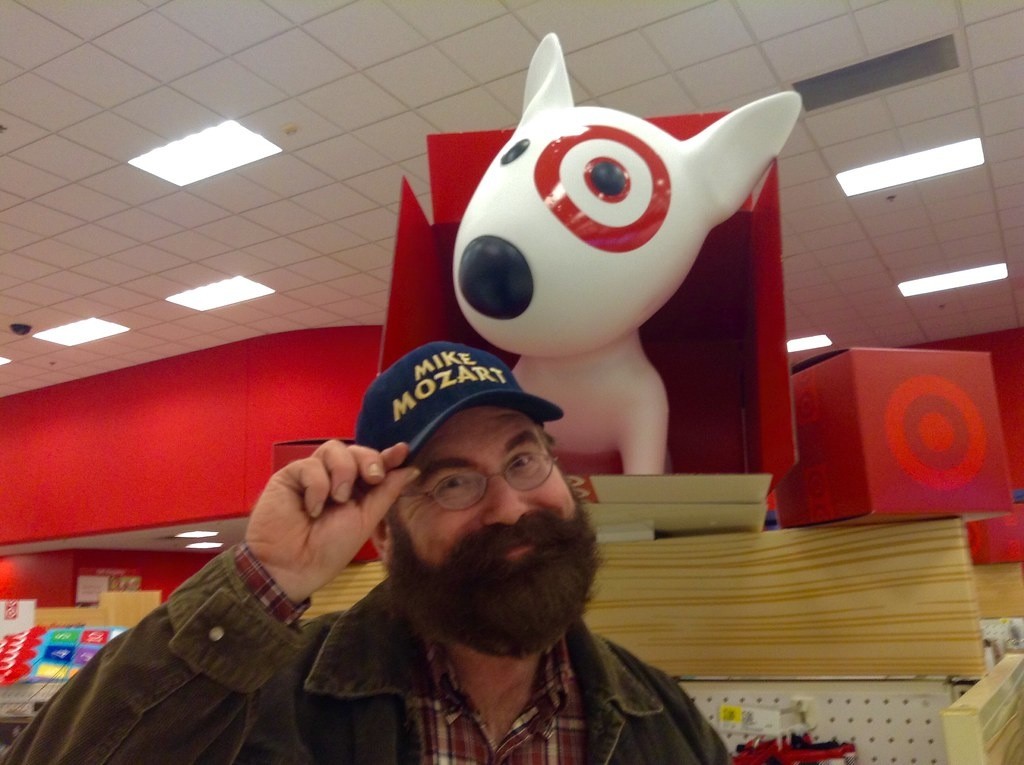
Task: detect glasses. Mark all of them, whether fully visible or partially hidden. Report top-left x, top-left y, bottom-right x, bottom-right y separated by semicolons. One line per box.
400;453;560;512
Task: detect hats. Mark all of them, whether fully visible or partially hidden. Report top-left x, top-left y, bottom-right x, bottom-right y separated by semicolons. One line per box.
355;341;564;468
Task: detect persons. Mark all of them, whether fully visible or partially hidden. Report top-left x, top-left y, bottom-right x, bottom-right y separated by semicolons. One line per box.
2;339;735;765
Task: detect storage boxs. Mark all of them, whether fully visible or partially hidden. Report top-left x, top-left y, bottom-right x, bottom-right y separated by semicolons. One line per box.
375;115;800;532
775;346;1014;527
965;503;1024;565
